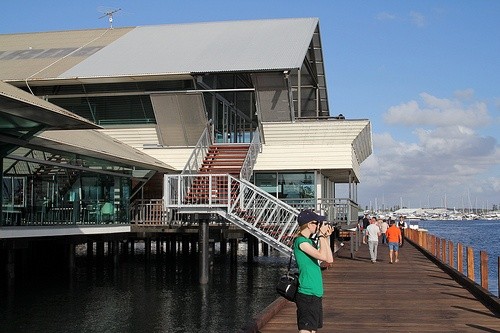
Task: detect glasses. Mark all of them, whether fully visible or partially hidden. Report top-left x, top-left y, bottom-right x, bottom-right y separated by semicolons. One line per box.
310;221;318;225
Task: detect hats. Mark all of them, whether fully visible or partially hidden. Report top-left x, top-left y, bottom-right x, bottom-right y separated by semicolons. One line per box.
297;210;326;226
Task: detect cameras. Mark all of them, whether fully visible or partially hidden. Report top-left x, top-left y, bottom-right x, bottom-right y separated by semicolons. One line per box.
320;221;340;238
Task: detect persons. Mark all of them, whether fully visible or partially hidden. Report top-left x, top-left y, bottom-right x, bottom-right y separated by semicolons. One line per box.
362;214;370;244
365;219;381;263
397;215;407;248
293;210;334;333
386;220;402;264
380;219;389;244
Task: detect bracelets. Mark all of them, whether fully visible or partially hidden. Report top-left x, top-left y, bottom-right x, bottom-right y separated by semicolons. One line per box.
318;236;323;240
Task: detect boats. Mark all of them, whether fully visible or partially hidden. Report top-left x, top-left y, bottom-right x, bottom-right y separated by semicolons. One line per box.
408;219;420;231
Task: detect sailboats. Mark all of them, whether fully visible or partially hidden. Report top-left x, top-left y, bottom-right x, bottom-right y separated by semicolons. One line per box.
368;189;500;221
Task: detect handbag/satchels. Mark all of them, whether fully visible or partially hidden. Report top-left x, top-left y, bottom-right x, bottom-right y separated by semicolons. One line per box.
276;273;299;302
